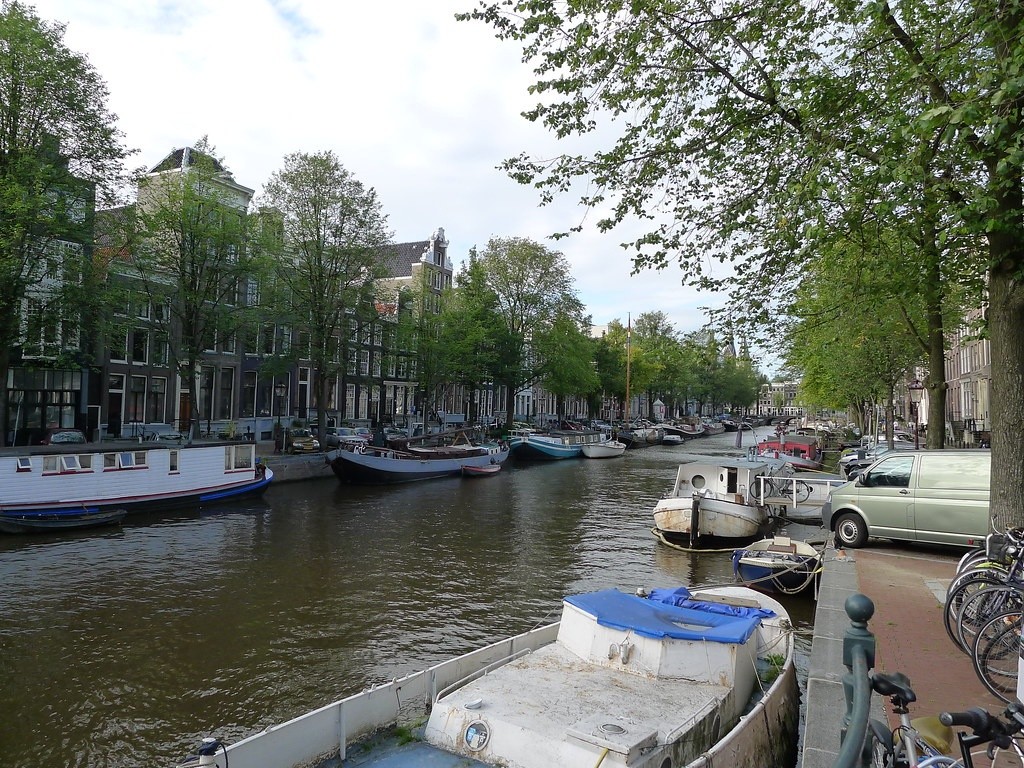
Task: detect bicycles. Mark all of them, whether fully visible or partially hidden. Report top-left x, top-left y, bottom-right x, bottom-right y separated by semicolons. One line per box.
859;672;1024;768
942;514;1024;705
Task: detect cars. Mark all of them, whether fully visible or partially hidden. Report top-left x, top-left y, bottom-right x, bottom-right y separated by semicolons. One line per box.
285;427;320;454
838;430;926;482
561;420;583;431
325;427;369;449
148;431;185;443
355;426;373;444
590;420;612;430
39;428;87;445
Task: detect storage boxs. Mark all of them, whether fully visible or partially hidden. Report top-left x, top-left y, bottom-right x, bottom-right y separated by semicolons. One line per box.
767;546;797;554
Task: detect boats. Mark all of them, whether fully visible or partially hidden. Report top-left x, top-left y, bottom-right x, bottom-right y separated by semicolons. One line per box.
617;415;835;472
323;425;510;485
460;463;502;477
581;439;627;459
508;428;612;461
0;440;275;517
170;585;798;768
732;536;822;596
653;456;788;553
0;508;128;536
662;434;686;444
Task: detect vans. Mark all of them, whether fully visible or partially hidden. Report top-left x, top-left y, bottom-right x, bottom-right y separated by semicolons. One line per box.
821;447;993;549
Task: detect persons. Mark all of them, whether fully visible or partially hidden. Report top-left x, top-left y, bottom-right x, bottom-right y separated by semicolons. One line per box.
274;434;281;454
7;428;19;447
881;417;885;431
894;419;898;430
